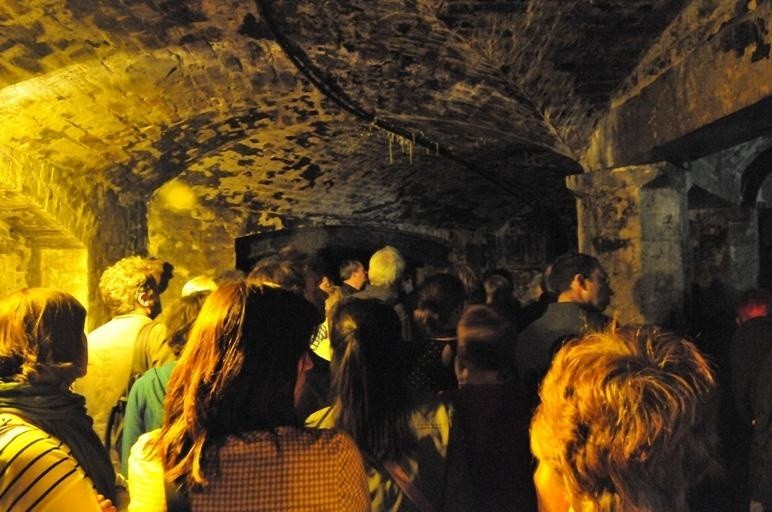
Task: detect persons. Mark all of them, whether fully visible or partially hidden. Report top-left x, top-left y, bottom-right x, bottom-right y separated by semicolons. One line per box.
531;325;716;512
0;241;619;512
717;287;771;511
